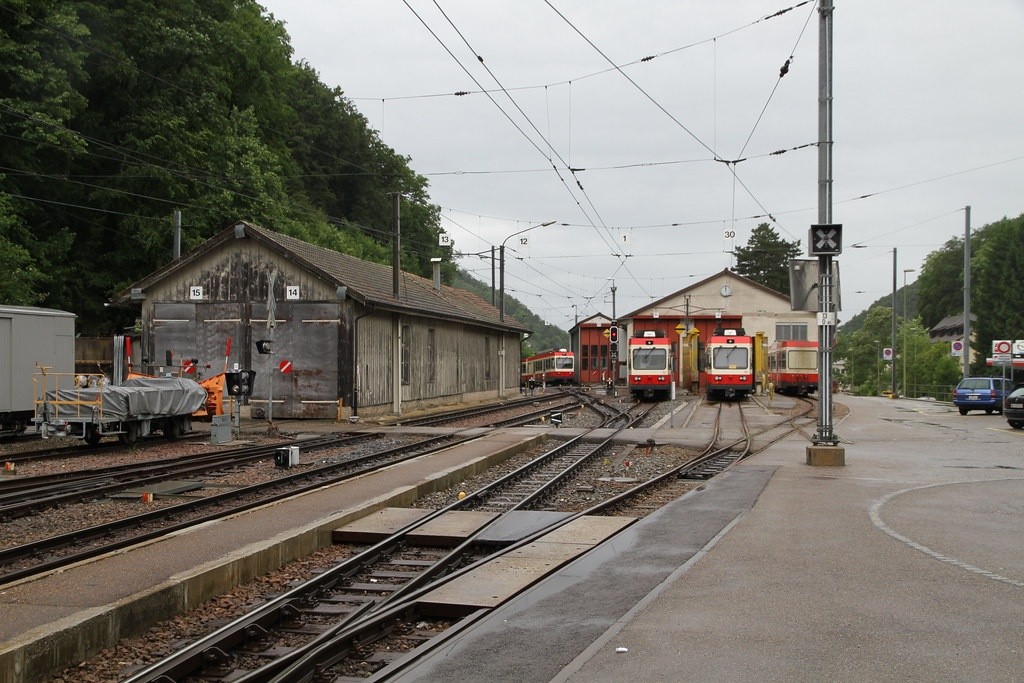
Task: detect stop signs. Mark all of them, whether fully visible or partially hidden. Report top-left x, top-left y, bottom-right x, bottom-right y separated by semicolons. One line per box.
279;360;293;374
183;360;196;373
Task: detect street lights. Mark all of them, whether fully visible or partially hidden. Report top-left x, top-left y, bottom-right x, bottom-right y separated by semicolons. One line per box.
610;326;618;344
903;268;916;398
847;348;855;386
873;340;881;396
500;219;558;322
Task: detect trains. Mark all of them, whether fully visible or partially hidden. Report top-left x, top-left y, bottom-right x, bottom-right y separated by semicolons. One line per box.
629;329;674;401
704;327;755;401
768;339;821;397
520;347;575;386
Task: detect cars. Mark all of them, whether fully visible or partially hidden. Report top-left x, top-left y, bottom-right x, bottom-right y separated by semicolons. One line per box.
1003;388;1024;430
953;376;1013;415
